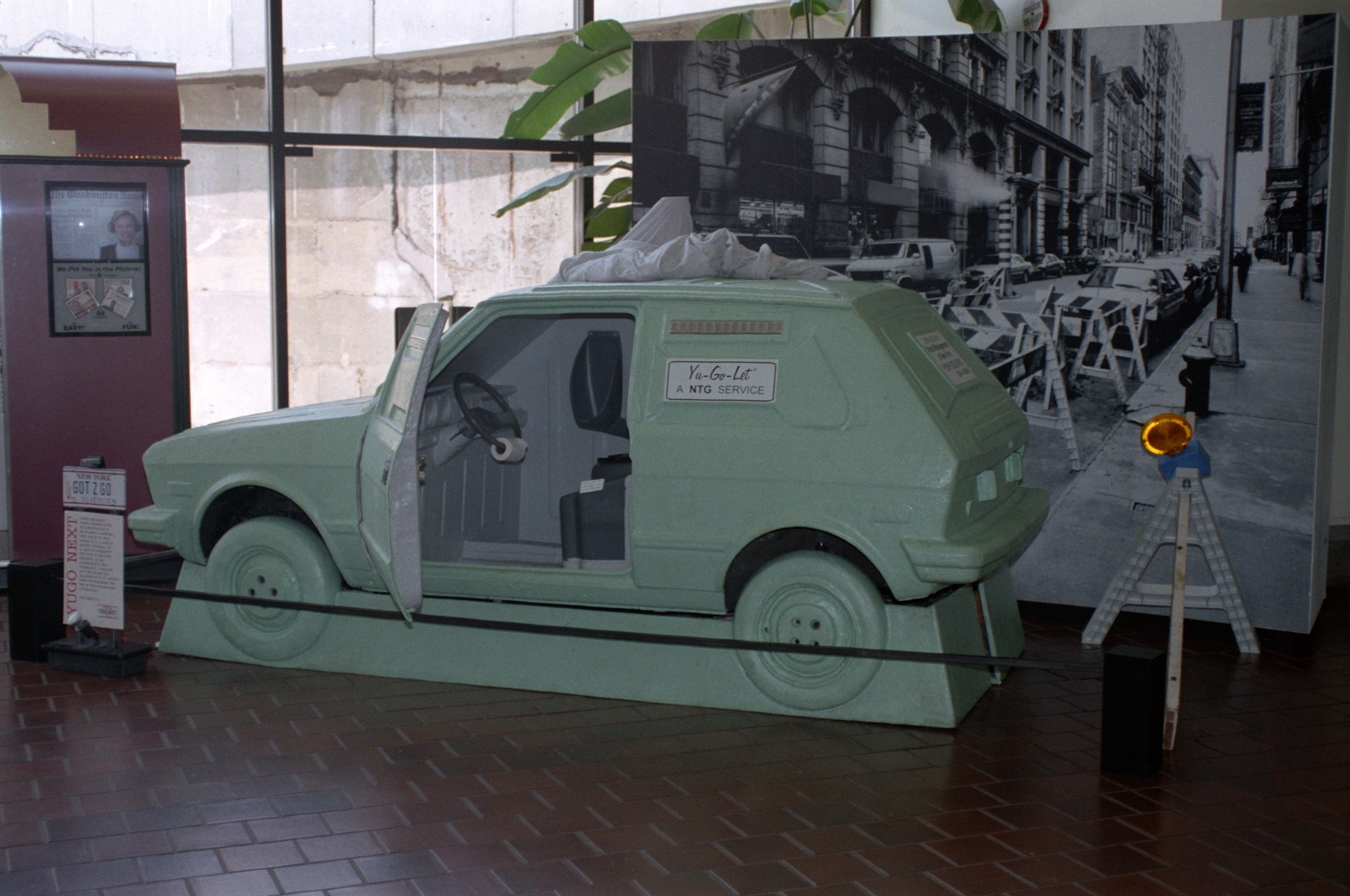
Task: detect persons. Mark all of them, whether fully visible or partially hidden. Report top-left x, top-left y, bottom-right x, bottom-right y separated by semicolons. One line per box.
1235;247;1252;294
99;209;144;260
1291;246;1320;302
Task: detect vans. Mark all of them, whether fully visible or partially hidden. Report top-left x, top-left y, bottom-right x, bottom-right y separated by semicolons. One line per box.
845;238;961;297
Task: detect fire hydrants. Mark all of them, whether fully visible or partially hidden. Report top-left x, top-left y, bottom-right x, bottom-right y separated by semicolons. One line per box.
1177;337;1216;416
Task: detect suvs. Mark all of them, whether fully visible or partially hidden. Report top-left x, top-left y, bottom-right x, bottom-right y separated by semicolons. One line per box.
695;226;811;264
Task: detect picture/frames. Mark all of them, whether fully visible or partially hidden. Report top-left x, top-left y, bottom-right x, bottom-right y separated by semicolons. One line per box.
44;181;152;337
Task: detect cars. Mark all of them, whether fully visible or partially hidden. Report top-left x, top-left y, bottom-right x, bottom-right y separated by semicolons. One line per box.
1054;251;1223;356
122;279;1056;713
965;247;1119;284
